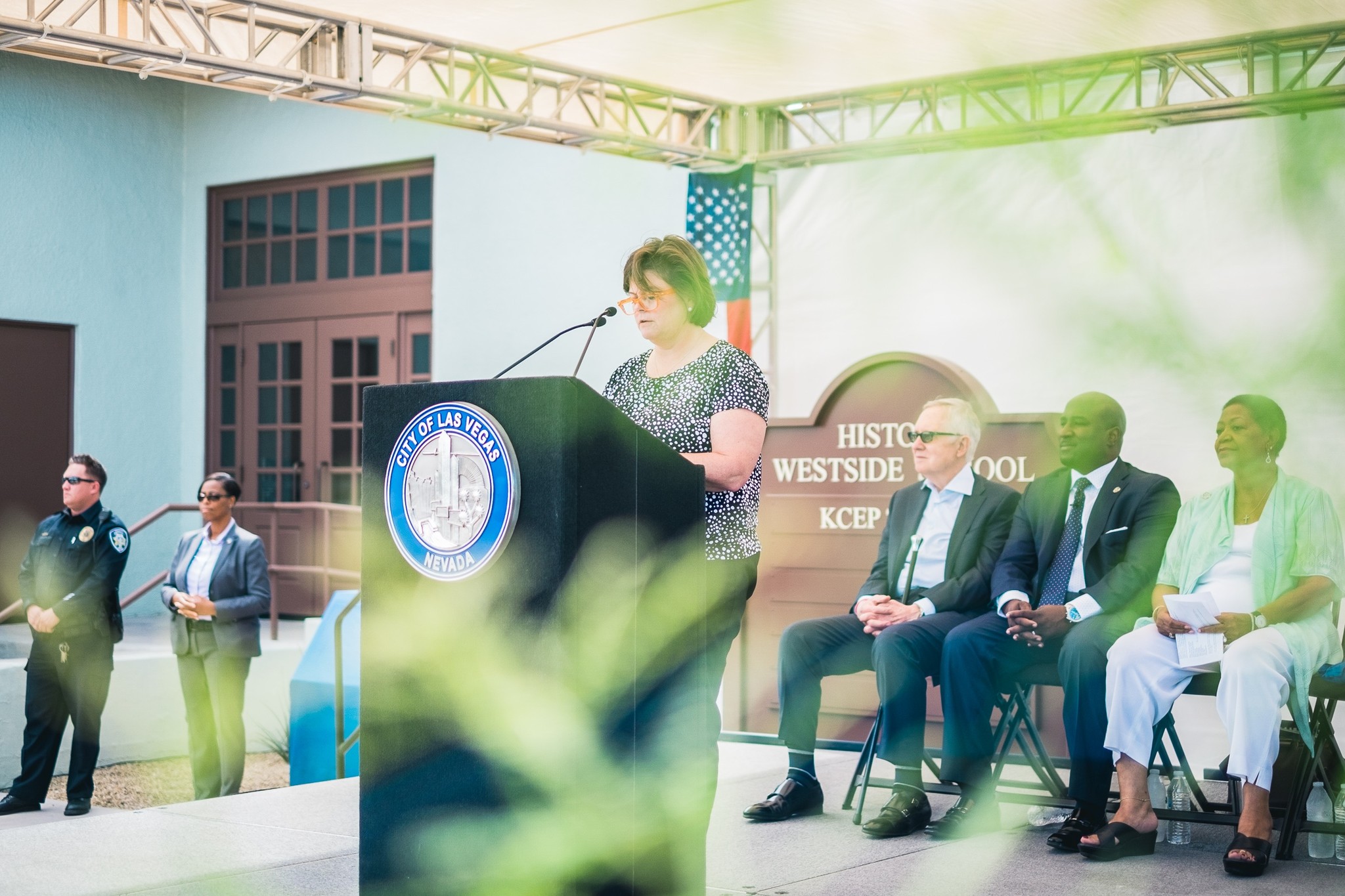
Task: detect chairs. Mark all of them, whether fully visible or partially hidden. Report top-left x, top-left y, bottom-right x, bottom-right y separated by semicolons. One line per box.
842;599;1345;861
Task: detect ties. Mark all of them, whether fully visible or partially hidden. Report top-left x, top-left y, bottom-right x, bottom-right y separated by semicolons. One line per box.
1037;478;1092;609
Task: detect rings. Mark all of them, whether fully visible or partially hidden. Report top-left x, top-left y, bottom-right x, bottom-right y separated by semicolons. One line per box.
1169;633;1172;638
1224;636;1227;643
1031;630;1036;635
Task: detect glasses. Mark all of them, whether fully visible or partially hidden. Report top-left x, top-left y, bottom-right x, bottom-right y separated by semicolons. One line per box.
907;431;963;443
198;492;230;501
617;288;675;316
62;477;94;485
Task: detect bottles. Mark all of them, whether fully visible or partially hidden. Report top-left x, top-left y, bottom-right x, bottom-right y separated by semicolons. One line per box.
1028;805;1074;826
1167;771;1191;844
1306;782;1334;858
1146;769;1166;842
1334;784;1345;860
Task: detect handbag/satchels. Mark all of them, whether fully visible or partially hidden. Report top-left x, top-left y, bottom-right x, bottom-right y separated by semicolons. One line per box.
1219;720;1345;821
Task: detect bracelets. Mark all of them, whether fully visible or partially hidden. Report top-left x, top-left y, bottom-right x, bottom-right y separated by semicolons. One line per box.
1152;605;1164;622
1249;613;1254;631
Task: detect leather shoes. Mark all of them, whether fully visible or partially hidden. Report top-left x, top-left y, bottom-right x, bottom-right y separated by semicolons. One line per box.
0;794;40;815
924;796;1002;837
862;793;931;836
742;780;823;820
64;797;91;815
1046;806;1109;851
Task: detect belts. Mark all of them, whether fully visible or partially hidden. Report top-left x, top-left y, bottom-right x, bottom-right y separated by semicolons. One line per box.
187;619;213;632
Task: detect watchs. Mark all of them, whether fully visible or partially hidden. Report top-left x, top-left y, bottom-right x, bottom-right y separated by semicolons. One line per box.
1254;611;1269;629
918;606;925;618
1064;604;1081;626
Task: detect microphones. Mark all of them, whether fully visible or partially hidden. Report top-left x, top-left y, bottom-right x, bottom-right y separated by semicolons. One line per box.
572;307;617;379
490;316;607;379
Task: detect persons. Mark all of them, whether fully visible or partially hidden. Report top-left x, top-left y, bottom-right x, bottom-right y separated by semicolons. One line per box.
160;472;271;800
1078;393;1345;876
0;454;131;815
601;235;768;896
924;392;1182;853
742;397;1022;836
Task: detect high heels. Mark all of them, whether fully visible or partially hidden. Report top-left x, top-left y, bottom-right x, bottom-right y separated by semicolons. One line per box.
1078;822;1158;860
1222;832;1272;876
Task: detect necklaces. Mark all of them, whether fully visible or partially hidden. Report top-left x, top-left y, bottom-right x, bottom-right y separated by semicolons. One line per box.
1234;474;1278;523
653;330;705;377
